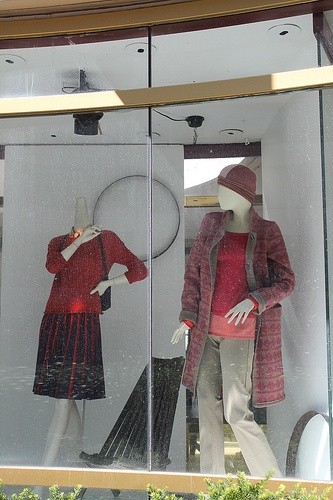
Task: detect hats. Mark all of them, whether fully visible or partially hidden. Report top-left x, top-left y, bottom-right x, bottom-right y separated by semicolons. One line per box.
217;164;257;204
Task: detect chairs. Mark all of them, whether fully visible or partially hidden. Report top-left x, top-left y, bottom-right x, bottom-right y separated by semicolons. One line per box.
79;356;186;500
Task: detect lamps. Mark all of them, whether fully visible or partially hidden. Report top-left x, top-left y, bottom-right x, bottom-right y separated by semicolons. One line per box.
61;70;104;136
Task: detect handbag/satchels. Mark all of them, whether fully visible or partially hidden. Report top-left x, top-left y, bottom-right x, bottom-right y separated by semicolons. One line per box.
98;235;111;311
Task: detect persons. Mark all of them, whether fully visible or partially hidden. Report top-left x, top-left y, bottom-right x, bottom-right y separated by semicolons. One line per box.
30;197;148;500
172;164;296;500
